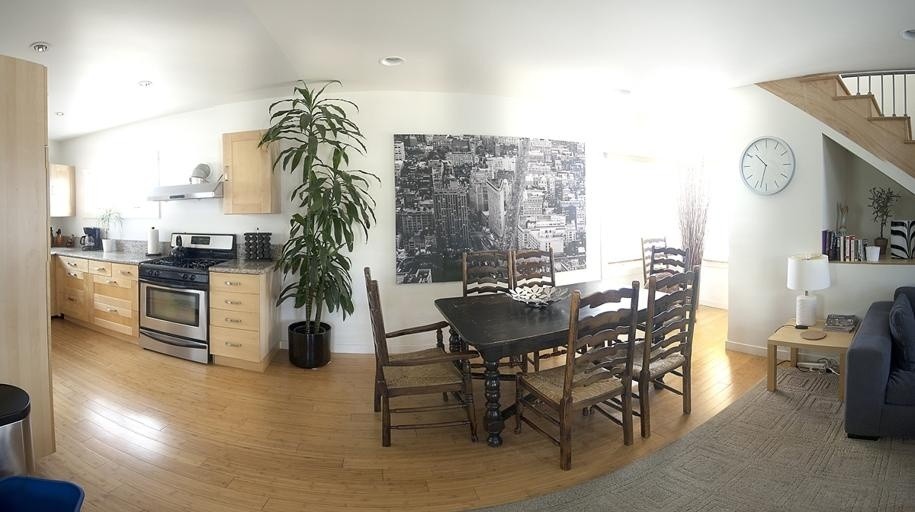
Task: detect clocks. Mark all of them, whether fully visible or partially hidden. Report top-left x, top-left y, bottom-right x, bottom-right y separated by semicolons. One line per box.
739;136;796;197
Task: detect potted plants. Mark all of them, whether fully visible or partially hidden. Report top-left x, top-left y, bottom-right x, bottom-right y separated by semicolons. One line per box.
256;78;381;369
867;186;902;256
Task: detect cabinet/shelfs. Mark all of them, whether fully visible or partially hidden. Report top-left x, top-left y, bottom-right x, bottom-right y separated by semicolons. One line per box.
221;128;283;216
210;260;280;373
51;253;88;330
88;259;139;348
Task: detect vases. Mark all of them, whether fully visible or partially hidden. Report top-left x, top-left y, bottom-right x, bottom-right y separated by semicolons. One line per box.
101;239;116;252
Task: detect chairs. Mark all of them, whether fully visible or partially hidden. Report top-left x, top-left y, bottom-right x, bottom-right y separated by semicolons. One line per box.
512;247;587;372
641;237;667;285
462;250;527;373
514;280;640;471
591;265;700;438
636;245;689;344
364;267;479;447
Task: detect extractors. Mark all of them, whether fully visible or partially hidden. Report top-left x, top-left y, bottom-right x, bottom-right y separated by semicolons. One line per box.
148;182;223;201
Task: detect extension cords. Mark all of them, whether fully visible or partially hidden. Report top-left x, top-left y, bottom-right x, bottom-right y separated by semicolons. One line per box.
797;361;826;370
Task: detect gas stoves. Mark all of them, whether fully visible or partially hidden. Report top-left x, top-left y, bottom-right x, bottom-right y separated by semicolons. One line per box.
146;256;226;272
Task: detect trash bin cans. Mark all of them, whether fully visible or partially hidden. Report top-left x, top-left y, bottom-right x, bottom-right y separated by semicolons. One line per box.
0;476;85;512
0;383;35;476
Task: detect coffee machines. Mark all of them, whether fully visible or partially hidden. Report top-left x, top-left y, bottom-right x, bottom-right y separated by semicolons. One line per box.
80;227;102;250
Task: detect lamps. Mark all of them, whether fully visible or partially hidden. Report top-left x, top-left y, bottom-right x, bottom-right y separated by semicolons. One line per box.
32;42;49;58
786;253;830;327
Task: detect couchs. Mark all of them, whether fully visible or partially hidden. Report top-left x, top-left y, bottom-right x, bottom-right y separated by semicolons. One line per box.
844;287;915;441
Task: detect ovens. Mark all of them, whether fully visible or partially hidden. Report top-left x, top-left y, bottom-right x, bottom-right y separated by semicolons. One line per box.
138;278;208;347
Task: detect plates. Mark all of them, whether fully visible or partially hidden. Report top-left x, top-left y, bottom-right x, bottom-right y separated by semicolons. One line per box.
801;331;826;340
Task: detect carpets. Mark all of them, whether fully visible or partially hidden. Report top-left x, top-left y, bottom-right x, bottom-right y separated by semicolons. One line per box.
461;359;915;511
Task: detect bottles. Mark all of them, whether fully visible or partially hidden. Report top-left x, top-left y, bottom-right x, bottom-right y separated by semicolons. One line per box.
67;240;73;248
55;228;64;246
50;227;54;247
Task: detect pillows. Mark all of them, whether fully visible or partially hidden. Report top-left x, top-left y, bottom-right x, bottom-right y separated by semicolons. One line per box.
888;294;914;370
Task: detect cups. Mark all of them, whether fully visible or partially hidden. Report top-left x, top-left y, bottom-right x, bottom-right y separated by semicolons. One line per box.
866;246;880;262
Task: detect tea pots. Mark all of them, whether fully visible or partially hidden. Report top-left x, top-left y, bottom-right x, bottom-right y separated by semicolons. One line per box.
171;236;190;259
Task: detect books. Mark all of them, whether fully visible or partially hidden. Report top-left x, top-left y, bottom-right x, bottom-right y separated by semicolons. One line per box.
824;314;856;334
821;229;865;263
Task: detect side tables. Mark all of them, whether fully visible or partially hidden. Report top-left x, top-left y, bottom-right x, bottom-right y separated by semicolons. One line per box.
767;319;855;402
